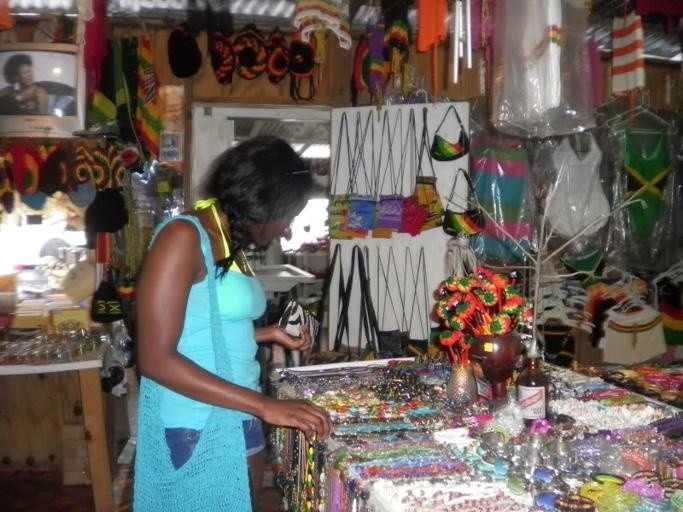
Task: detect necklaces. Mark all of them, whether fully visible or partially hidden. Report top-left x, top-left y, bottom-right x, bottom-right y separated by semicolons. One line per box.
220;217;246;273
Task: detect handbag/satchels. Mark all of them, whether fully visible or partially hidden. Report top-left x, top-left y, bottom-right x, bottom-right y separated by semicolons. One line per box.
443;208;485;236
328;175;446;238
134;354;251;512
306;330;429;364
431;128;470;160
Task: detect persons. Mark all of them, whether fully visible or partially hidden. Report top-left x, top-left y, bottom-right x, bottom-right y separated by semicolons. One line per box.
0;55;50;115
132;134;334;512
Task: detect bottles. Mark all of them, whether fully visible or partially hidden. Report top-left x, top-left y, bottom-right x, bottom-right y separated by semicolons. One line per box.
516;357;549;425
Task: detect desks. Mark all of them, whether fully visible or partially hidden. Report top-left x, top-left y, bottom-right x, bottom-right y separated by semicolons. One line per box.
0;328;140;511
265;356;683;512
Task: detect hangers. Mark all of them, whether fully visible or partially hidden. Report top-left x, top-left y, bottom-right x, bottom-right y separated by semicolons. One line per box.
533;256;683;335
593;90;679;137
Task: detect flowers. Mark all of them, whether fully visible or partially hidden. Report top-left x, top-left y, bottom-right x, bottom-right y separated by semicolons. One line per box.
429;267;536;363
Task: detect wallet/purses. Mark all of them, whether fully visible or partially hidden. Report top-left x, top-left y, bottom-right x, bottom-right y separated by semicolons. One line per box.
278;298;321;340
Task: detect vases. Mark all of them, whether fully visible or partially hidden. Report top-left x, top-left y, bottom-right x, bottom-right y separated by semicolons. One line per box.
447;332;523;408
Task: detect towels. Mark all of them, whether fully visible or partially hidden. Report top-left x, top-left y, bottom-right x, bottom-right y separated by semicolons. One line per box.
610;12;646;96
